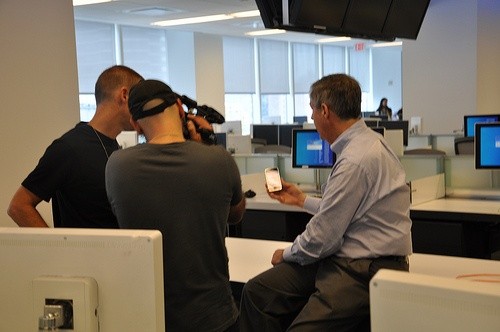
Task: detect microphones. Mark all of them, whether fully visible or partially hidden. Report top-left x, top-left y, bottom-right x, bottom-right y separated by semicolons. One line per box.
197;105;225;125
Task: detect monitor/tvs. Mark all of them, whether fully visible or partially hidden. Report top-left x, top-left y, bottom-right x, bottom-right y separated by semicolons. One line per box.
0;226;168;332
369;269;500;332
361;111;500;170
218;121;337;169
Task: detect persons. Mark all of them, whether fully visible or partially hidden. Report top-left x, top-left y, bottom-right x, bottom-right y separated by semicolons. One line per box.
105;80;246;332
239;74;412;332
377;98;392;118
7;65;145;230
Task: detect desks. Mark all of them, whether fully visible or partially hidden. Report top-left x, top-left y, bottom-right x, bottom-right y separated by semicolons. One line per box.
224;238;498;290
410;195;500;215
245;183;325;214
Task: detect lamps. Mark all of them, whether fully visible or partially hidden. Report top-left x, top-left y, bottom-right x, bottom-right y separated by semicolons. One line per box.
152;9;404;47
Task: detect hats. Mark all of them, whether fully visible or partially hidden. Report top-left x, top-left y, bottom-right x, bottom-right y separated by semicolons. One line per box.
128;80;177;120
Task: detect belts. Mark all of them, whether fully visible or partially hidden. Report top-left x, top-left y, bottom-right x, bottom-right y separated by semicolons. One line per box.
380;255;407;263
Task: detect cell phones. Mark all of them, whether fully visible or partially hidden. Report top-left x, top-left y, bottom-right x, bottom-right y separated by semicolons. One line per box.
265;167;282;193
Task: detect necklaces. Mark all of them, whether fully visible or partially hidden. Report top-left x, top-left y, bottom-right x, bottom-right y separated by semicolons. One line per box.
91;124;109;159
151;133;184;141
